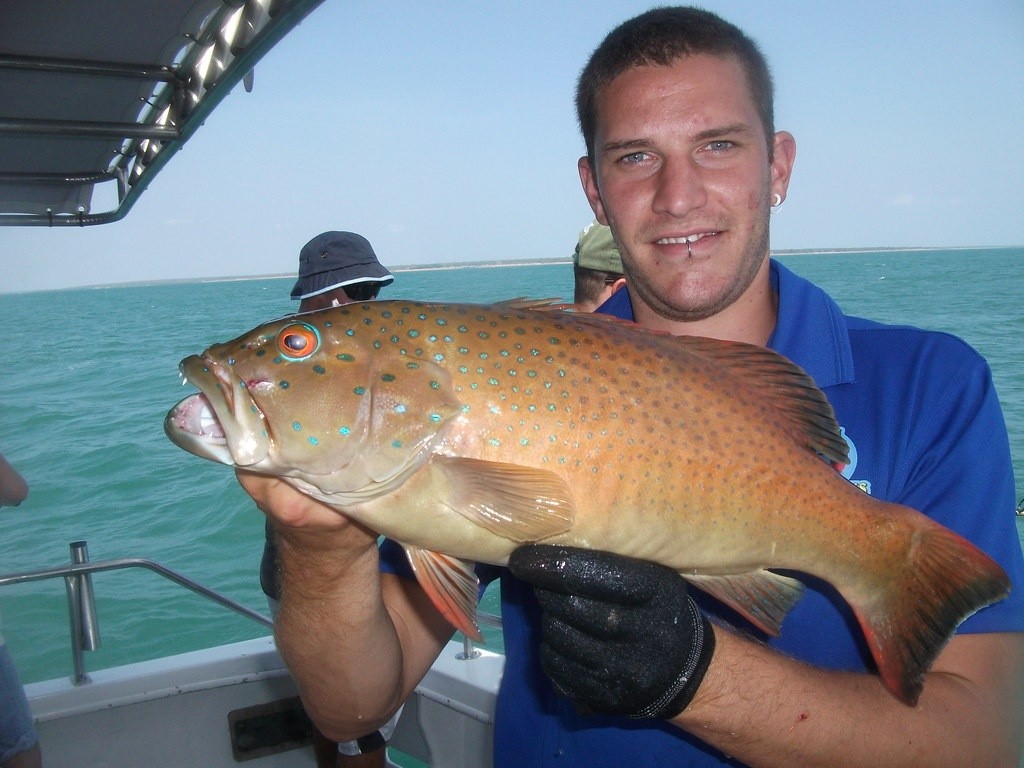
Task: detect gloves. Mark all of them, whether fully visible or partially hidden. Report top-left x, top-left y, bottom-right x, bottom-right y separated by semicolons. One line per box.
505;544;715;733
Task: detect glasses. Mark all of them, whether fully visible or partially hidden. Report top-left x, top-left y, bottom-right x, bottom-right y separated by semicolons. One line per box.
342;281;383;300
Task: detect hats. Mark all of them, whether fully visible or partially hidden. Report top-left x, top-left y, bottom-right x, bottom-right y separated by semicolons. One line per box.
572;219;648;274
291;231;394;301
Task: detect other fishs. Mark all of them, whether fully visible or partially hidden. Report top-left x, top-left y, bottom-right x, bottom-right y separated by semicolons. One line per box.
163;293;1017;711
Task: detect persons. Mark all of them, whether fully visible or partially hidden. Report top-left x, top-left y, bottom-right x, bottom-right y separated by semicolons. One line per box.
260;230;408;768
572;219;628;312
1;452;43;768
235;4;1023;768
